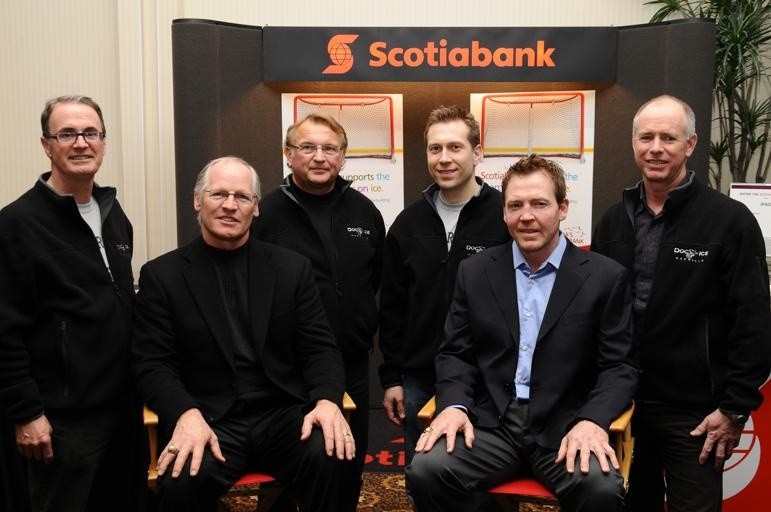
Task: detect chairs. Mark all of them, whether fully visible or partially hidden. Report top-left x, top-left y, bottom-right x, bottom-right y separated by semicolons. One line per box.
144;392;355;512
417;393;636;511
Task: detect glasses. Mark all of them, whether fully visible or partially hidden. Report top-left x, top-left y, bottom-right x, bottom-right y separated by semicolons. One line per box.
288;141;345;156
45;128;106;144
201;187;260;205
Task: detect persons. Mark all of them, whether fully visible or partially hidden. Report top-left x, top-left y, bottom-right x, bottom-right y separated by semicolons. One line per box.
403;153;644;512
588;93;770;512
378;105;511;512
128;156;362;512
0;94;151;512
249;110;386;511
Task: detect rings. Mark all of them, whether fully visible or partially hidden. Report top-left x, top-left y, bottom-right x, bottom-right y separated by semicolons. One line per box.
168;445;179;455
343;432;350;436
423;426;434;434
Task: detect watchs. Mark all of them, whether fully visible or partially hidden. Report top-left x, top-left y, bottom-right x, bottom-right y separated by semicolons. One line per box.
724;413;747;424
